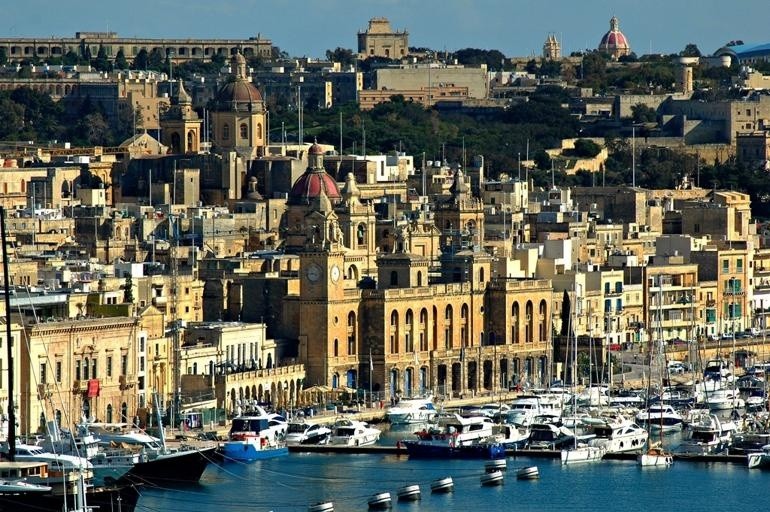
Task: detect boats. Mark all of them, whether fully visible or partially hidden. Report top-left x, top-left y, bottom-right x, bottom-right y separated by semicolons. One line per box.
221;397;291;468
